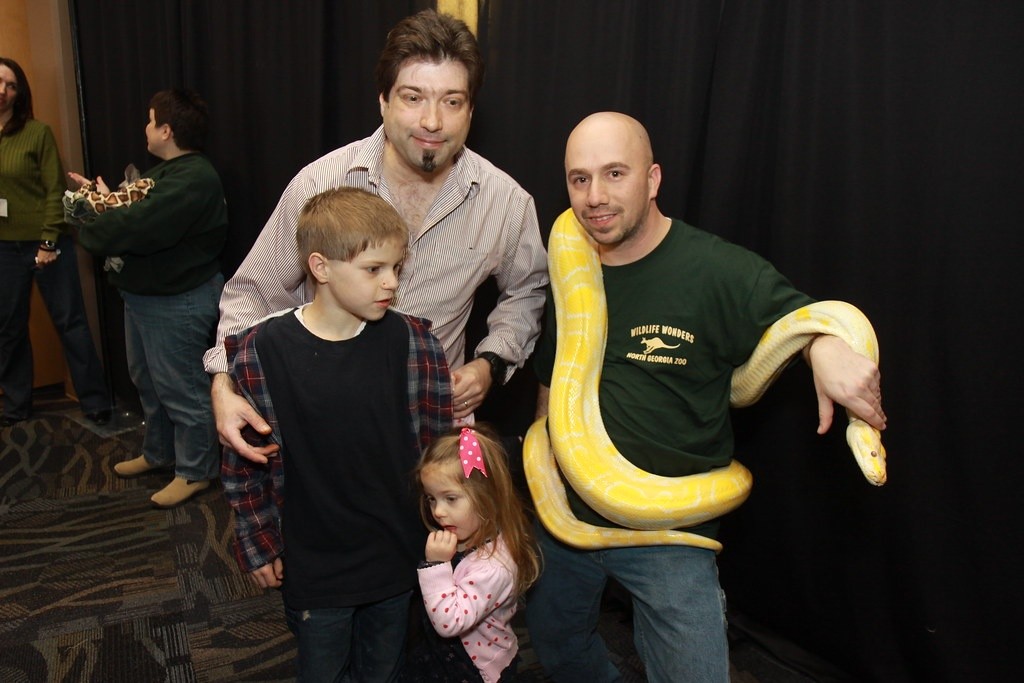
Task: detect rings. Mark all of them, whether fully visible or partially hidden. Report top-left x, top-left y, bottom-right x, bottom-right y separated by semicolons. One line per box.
464;401;468;409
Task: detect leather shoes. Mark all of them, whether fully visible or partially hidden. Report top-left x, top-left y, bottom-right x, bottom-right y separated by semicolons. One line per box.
0;416;29;427
113;454;156;477
150;477;210;510
90;409;111;425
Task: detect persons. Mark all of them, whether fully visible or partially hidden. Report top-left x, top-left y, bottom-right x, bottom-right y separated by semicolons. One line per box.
416;427;543;683
69;88;230;509
1;56;110;427
222;188;456;683
523;112;889;683
203;10;552;463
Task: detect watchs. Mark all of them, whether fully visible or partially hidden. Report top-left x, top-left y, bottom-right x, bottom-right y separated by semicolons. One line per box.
41;240;57;249
478;351;506;388
